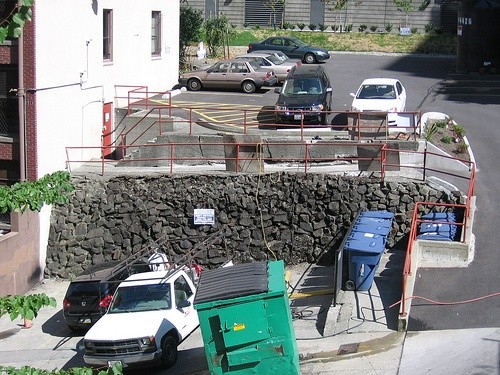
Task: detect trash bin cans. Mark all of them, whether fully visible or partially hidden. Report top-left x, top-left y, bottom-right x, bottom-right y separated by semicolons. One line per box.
344;210;395;291
417;212;456;242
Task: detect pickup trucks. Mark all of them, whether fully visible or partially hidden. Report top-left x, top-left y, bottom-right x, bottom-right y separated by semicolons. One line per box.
81;257;234;368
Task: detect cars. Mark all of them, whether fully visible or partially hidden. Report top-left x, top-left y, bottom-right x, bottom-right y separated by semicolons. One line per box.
249;50;302;66
232;54;297;85
249;36;331;64
350;78;407;114
178;59;272;94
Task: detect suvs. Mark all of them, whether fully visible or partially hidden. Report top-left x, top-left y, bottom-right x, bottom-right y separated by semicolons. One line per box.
62;261;151;330
274;65;333;126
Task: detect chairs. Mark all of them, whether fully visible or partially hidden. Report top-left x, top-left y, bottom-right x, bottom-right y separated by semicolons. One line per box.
288;82;302;94
165;290;187;308
